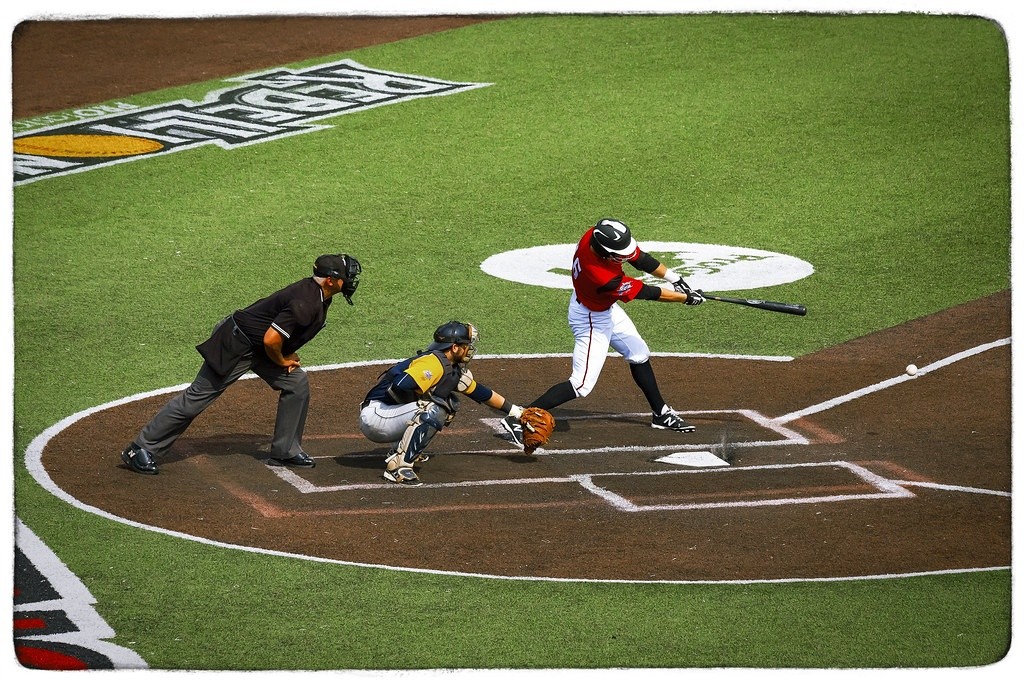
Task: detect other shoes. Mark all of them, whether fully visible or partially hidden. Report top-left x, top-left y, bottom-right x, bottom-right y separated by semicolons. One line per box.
121;442;159;475
267;451;315;469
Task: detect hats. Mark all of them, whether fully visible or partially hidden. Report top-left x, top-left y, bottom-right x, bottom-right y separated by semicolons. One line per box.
314;254;349;278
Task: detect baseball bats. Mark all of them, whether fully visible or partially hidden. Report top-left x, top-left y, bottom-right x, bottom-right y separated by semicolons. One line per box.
702;294;807;316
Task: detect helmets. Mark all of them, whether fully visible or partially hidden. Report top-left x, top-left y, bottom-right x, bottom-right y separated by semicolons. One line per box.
427;321;476;350
593;218;637;256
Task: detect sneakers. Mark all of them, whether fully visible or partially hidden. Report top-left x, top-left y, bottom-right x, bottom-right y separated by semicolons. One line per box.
382;464;423;487
650;403;697;433
388;446;430;462
499;414;524;445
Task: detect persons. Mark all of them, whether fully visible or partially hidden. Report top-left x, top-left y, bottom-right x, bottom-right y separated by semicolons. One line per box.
119;253;362;475
358;321;543;486
500;218;706;449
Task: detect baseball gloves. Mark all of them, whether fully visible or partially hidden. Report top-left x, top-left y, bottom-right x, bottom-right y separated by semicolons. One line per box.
520;406;557;457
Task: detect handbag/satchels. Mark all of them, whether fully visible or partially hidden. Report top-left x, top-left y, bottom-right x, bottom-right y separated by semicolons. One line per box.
195;315;254;379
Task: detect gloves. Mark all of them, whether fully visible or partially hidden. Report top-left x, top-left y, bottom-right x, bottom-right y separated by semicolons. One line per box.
671;276;692;295
684;289;706;306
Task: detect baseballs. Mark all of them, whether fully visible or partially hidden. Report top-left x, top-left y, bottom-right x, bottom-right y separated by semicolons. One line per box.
906;362;920;377
